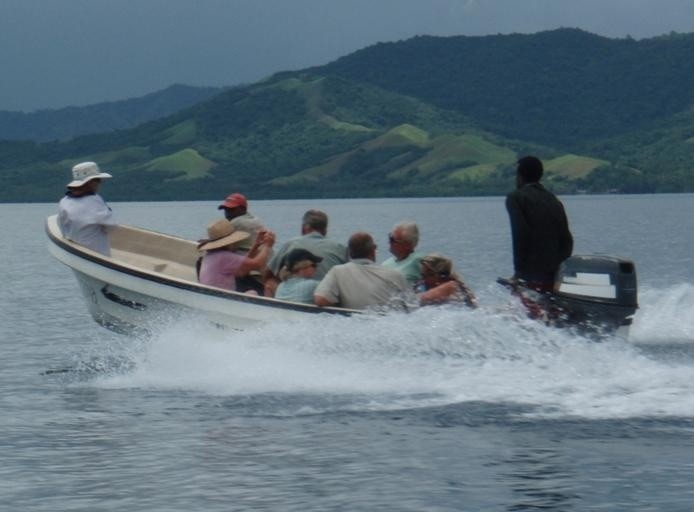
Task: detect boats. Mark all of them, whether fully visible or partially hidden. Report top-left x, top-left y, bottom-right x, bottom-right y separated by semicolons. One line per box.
43;212;640;341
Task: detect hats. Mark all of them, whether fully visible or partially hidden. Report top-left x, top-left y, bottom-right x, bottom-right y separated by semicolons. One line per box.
283;246;325;271
196;219;250;253
418;251;454;277
216;193;249;211
66;162;112;187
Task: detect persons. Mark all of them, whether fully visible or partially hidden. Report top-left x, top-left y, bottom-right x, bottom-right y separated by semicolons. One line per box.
197;192;477;313
505;155;573;292
56;161;119;258
